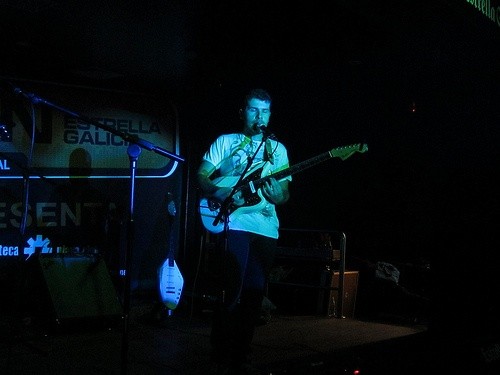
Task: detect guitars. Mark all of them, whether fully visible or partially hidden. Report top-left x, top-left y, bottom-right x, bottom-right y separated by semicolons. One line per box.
198;143;369;234
157;189;185;312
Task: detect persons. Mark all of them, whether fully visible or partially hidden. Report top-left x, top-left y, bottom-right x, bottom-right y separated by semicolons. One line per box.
198;89;294;375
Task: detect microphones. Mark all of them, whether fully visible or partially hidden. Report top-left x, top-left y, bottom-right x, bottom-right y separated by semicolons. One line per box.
254;123;276;139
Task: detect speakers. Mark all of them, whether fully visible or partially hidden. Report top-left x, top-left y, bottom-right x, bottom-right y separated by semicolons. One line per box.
269;256;336;319
